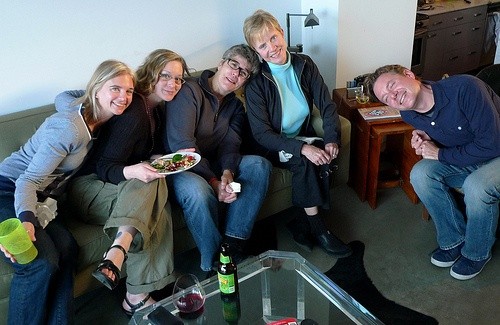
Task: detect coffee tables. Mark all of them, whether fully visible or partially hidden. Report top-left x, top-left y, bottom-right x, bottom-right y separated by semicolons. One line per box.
128;251;384;325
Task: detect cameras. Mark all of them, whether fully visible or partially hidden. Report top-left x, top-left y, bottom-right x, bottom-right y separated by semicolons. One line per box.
346;87;362;98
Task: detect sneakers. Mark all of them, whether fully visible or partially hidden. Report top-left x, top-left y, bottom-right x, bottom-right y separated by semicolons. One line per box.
449;256;491;280
430;238;466;268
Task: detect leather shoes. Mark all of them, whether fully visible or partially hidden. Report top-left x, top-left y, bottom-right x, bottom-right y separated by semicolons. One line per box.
286;218;315;252
315;230;352;258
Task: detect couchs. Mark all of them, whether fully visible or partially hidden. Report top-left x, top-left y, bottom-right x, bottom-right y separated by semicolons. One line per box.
0;68;351;325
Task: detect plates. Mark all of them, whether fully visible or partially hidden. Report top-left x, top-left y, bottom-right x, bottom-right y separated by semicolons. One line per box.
150;152;201;174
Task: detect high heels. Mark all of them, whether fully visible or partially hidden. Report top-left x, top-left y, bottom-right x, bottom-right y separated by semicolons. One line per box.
91;245;128;290
121;293;151;319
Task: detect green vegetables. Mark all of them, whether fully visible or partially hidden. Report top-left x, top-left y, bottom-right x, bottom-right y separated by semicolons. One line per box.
150;154;183;169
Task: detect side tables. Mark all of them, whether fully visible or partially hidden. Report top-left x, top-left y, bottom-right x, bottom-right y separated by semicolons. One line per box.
334;88;422;209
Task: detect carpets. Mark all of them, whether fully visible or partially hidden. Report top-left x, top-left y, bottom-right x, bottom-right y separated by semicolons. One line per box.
71;224;439;325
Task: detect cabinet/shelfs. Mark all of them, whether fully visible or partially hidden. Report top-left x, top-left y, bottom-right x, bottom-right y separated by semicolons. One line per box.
420;5;488;77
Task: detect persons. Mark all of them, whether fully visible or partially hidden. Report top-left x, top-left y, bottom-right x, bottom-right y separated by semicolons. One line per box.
368;64;500;281
162;43;272;279
243;8;354;258
65;49;184;325
0;59;135;325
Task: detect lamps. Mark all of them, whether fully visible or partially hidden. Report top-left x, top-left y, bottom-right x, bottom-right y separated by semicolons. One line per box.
286;9;319;54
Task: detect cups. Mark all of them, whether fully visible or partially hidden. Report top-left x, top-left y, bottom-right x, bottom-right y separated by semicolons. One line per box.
173;274;205;313
0;218;38;264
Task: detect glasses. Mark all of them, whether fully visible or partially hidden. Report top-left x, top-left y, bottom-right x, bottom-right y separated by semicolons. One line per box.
158;73;186;85
223;58;251;80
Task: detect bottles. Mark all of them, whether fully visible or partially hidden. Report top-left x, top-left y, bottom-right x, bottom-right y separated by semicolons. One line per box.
221;293;242;325
218;243;238;297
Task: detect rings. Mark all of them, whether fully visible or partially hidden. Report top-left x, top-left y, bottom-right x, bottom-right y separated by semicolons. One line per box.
332;154;335;157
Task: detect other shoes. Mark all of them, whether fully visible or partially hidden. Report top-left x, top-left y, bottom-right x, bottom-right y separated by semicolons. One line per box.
211;242;241;273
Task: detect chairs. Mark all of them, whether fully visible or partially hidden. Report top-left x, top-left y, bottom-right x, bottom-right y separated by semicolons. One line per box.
423;63;500;220
